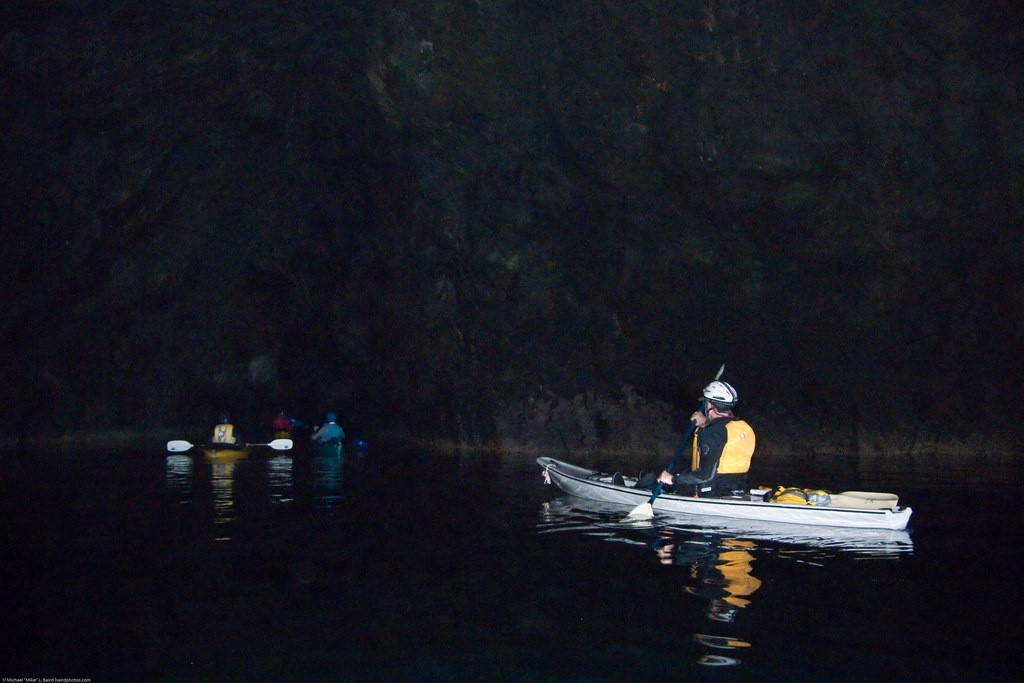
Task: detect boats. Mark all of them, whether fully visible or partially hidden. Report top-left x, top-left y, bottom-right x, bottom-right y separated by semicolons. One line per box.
271;429;292;441
202;446;253;462
535;454;913;533
314;440;343;459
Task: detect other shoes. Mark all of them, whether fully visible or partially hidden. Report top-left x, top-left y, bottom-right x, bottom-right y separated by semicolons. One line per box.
612;472;625;486
639;470;648;480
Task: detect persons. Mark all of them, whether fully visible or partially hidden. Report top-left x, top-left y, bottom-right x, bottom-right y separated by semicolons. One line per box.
658;382;756;498
213;410;345;447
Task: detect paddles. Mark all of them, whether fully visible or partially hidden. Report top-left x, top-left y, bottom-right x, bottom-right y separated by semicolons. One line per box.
288;418;368;448
622;364;726;521
167;438;294;451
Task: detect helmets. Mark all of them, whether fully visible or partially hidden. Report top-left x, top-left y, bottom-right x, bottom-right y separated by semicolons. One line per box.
703;381;738;405
278;411;285;416
218;410;232;423
325;412;336;422
